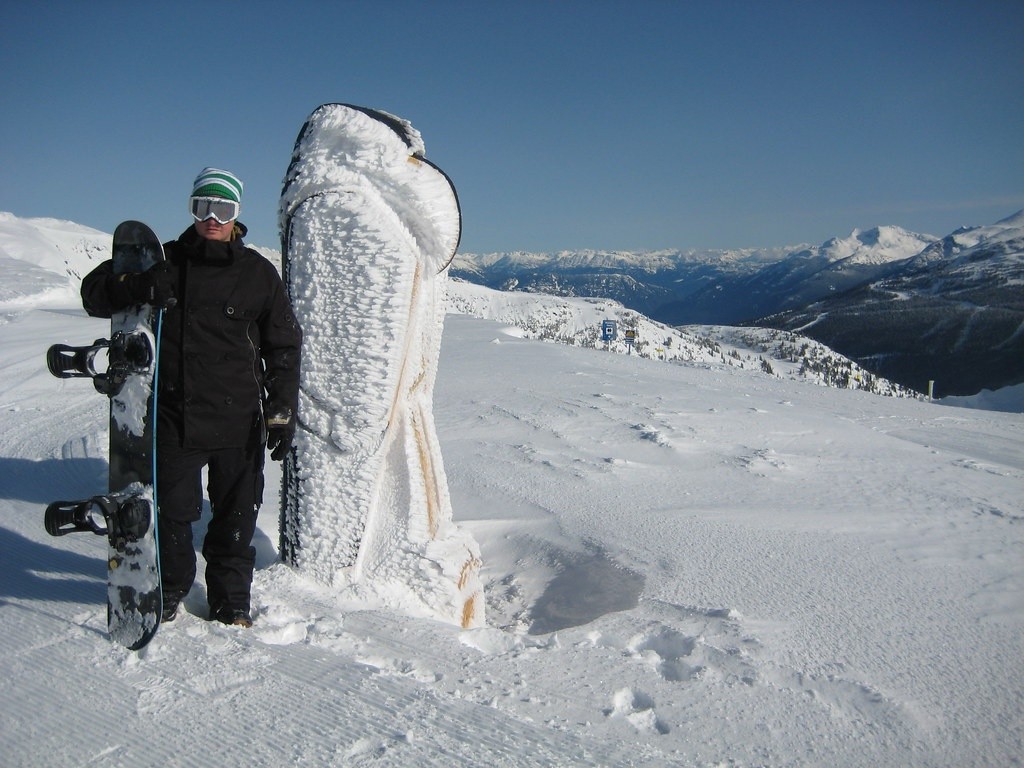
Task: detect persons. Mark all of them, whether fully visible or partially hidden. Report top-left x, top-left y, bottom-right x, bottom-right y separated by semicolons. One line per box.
79;166;304;628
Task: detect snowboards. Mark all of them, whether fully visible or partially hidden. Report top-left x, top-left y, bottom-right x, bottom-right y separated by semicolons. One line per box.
42;219;164;651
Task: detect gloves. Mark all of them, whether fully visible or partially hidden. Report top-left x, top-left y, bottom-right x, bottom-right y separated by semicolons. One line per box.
267;405;299;461
135;261;177;309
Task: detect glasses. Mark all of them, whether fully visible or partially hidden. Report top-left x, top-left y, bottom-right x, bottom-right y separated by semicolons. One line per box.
189;196;241;225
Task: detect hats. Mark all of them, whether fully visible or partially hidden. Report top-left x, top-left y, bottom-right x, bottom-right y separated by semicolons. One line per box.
190;167;244;203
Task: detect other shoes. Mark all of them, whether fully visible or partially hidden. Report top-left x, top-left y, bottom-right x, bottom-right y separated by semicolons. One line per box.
162;590;184;622
209;605;253;628
